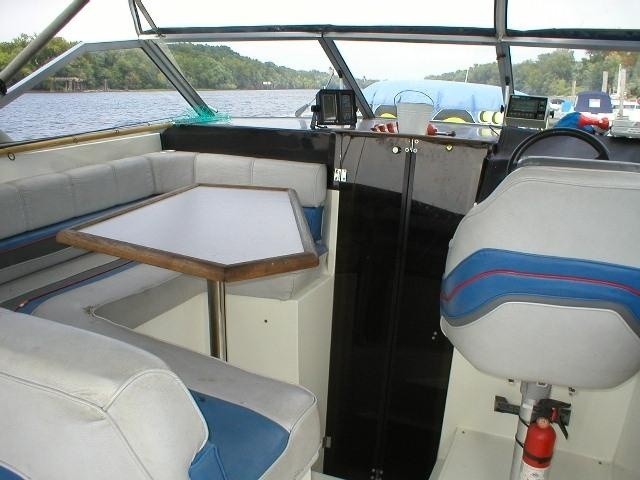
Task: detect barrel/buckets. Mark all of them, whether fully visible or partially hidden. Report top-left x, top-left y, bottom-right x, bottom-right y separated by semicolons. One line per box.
393;90;435;135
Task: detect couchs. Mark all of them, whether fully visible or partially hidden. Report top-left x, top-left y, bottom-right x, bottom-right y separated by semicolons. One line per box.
0;149;329;480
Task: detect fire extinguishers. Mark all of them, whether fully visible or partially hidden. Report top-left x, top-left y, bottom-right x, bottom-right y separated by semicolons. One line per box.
517;398;571;480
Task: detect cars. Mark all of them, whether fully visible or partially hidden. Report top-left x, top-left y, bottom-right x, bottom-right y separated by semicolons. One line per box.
548;90;640;136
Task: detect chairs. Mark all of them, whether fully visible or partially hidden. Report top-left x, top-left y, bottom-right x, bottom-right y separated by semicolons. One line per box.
429;156;640;480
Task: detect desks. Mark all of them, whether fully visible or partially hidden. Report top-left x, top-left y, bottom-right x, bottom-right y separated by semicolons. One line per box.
55;183;320;360
